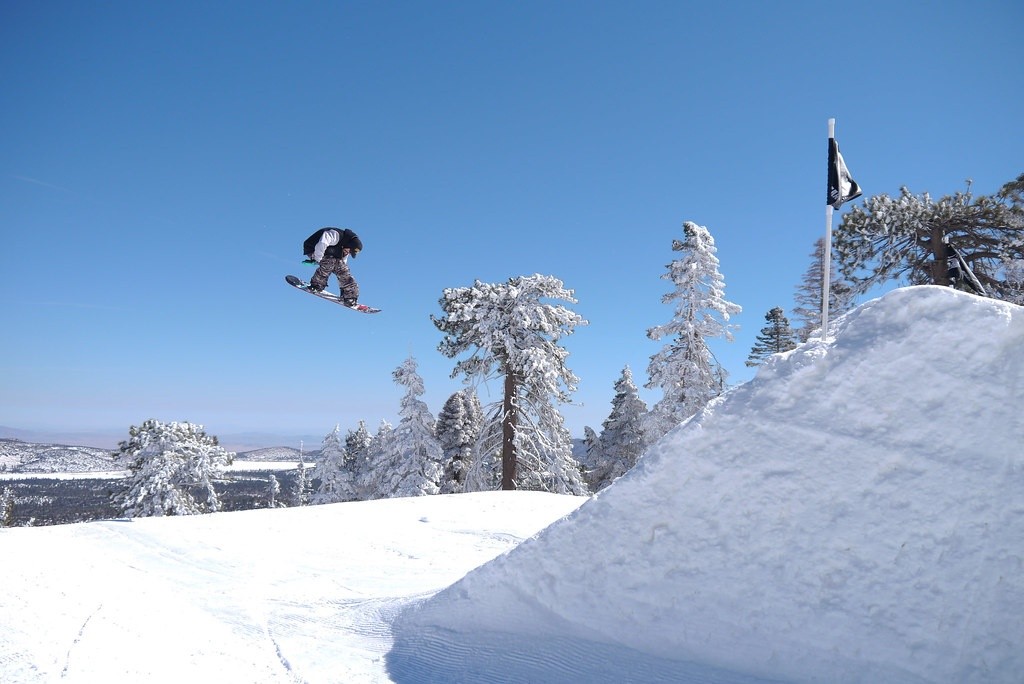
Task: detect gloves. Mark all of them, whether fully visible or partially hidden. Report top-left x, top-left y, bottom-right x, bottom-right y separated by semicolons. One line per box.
302;259;316;263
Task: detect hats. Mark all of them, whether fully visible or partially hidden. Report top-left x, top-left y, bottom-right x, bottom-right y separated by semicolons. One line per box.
350;237;363;259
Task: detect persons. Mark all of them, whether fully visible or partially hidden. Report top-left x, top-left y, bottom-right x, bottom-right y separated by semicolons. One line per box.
303;227;363;306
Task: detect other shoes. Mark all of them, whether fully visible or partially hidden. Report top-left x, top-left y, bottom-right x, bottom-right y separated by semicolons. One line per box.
307;283;324;293
343;298;358;307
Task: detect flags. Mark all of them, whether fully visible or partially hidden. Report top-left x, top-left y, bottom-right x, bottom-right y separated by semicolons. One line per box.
826;138;862;209
944;239;988;297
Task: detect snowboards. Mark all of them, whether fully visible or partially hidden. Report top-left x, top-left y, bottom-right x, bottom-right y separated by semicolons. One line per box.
285;275;382;314
